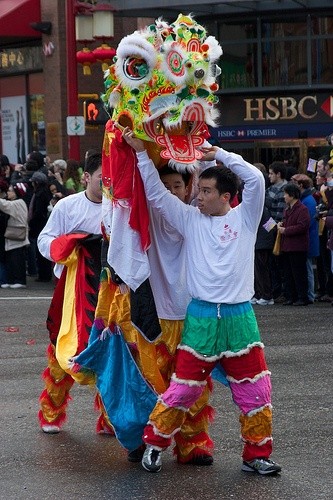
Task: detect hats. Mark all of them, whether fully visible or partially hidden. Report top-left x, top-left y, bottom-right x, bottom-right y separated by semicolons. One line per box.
12;182;28;197
28;172;47;182
53;159;67;171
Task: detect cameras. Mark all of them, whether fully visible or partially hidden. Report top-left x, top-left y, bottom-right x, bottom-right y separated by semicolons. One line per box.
314;204;329;222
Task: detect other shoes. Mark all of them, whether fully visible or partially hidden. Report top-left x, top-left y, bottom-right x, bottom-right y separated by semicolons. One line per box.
1;284;26;289
255;298;275;305
282;300;295;305
127;448;142;462
250;298;258;305
190;455;214;465
42;424;60;434
293;301;307;306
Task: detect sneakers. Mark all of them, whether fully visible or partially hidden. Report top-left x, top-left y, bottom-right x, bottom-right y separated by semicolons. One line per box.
241;457;282;476
142;445;164;472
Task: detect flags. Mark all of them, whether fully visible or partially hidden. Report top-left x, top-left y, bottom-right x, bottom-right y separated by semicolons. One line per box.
307;159;317;172
262;217;277;232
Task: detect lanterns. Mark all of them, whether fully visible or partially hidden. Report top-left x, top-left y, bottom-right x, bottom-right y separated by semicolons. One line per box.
76;48;96;75
93;43;116;72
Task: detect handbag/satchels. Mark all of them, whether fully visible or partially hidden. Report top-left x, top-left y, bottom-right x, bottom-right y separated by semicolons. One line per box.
5;225;27;241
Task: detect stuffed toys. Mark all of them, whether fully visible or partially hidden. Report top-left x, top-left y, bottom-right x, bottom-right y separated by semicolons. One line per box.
56;13;223;461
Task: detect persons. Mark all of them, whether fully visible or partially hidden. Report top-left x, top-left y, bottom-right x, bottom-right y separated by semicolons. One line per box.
127;163;213;466
121;126;282;475
217;133;333;306
0;150;87;289
37;152;115;435
